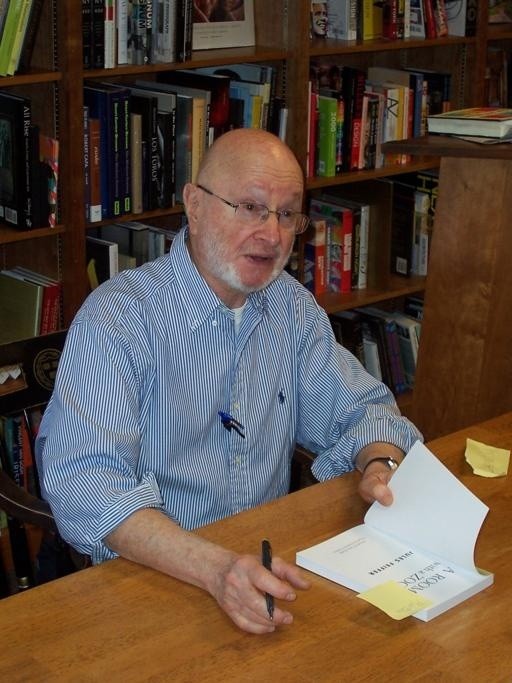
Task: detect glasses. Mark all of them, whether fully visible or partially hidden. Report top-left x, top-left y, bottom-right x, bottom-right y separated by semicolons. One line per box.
195;183;310;235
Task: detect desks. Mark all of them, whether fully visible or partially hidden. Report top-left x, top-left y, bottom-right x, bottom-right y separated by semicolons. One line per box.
0;411;512;682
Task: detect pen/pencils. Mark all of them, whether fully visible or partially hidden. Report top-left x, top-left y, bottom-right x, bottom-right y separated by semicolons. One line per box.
262;539;274;619
218;410;244;439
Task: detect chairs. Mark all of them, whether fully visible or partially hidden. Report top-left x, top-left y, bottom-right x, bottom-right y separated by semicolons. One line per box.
1;399;320;592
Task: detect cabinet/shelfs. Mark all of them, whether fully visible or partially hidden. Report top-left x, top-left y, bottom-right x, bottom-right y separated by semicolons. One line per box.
0;0;512;597
380;129;512;445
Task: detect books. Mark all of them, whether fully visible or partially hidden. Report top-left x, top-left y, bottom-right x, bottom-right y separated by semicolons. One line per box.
1;1;288;487
296;440;493;623
309;0;510;41
305;44;512;171
301;169;441;298
328;296;423;397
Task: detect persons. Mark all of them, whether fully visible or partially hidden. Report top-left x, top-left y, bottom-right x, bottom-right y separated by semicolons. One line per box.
35;127;424;634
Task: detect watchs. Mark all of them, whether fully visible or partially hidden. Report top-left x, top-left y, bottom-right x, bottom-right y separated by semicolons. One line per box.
363;456;399;471
